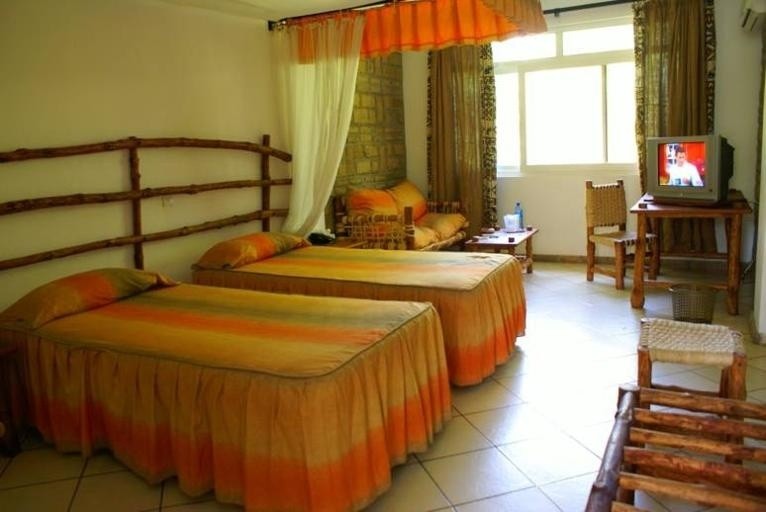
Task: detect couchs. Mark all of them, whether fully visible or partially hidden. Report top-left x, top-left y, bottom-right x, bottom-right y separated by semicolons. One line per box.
332;195;469;251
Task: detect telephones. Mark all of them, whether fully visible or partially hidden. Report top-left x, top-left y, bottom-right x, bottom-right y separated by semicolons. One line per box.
308;233;335;245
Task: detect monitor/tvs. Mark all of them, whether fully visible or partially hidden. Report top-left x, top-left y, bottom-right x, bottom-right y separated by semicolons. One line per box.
646;133;734;205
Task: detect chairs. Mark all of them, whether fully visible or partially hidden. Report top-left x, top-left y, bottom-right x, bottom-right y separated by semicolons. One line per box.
585;179;660;289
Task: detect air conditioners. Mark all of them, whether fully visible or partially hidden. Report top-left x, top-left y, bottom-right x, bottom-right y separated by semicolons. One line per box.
738;0;766;32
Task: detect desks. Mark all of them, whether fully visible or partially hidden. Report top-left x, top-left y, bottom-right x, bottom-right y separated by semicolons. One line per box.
629;189;753;315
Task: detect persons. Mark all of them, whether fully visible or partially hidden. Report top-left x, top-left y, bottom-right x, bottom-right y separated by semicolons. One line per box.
668;147;702;186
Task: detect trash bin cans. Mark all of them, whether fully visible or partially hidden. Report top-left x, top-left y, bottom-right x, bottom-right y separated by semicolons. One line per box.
668;283;719;324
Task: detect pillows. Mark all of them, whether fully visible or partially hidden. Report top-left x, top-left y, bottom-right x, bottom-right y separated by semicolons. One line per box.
191;232;312;270
0;268;181;332
346;185;398;215
385;178;428;221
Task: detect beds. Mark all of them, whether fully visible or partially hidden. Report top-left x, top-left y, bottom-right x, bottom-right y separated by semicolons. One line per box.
193;245;527;387
0;284;454;512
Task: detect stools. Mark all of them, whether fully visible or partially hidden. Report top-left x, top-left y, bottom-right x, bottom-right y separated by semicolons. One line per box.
637;317;747;463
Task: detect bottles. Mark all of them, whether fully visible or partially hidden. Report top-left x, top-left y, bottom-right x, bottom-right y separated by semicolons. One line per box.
513;201;523;230
